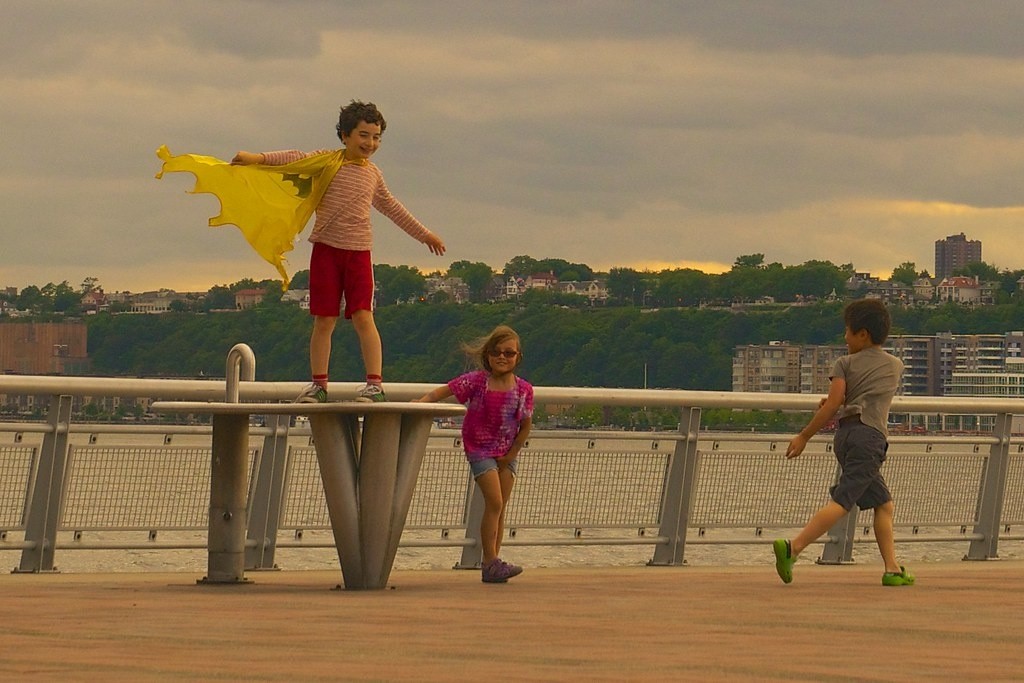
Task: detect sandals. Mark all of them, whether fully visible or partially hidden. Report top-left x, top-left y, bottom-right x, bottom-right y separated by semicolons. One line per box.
883;565;913;586
773;539;797;583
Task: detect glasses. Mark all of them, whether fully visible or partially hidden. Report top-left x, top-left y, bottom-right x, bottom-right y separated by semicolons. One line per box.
489;349;519;358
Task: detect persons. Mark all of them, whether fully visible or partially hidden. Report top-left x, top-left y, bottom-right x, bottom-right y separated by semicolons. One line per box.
772;298;917;586
409;326;534;584
229;101;447;403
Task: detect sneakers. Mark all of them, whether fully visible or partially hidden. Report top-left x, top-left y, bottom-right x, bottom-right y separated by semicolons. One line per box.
294;384;328;404
480;559;522;583
356;384;386;402
